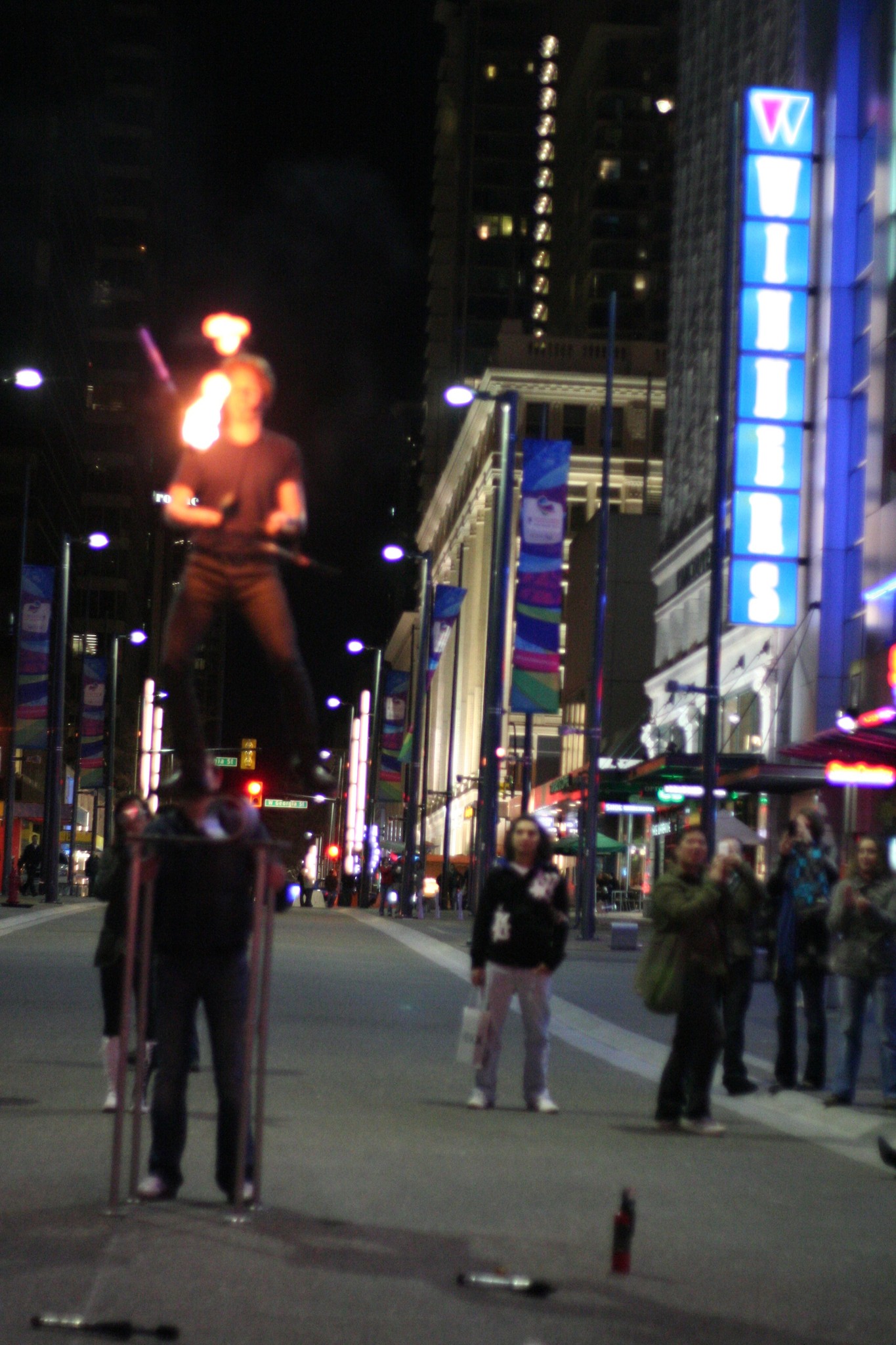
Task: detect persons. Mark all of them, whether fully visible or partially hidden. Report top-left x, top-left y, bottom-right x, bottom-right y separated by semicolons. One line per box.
159;354;319;794
596;871;620;904
324;867;369;908
435;864;468;921
85;848;99;896
468;816;569;1112
636;806;896;1136
380;855;427;919
298;864;313;907
18;835;41;896
89;796;264;1204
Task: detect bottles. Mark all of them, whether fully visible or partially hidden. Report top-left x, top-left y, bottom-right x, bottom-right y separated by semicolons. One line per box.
609;1188;634;1274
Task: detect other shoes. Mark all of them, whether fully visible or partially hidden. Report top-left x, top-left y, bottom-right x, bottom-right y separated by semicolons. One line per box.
137;1178;177;1198
824;1093;852;1106
101;1092;117;1113
130;1093;149;1115
468;1092;494;1110
527;1097;559;1113
155;767;214;795
730;1083;757;1095
799;1078;826;1091
680;1114;727;1136
768;1082;797;1092
232;1180;254;1204
297;763;339;795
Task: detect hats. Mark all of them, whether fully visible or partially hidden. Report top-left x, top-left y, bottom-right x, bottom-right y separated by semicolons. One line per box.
222;352;276;410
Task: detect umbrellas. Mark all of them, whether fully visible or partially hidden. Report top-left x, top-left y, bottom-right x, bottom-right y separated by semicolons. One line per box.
584;831;626;912
550;832;586;886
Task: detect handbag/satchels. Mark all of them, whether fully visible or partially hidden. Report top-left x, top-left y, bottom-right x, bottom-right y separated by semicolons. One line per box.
635;930;689;1015
455;985;489;1070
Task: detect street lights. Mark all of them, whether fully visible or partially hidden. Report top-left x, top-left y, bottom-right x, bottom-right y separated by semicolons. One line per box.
326;695;355;906
312;749;344;907
40;528;112;909
101;627;148;899
445;380;522;943
345;638;382;910
380;543;435;918
